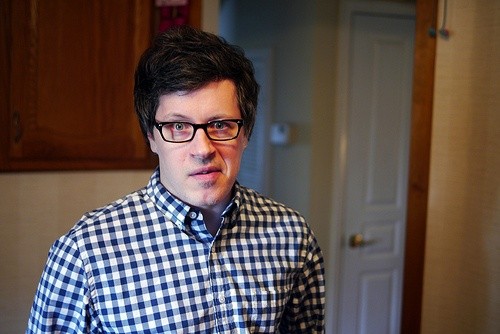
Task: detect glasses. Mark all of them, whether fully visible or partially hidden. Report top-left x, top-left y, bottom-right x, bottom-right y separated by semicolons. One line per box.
151;119;245;143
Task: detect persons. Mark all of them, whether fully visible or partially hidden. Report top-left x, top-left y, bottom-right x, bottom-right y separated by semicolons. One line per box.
25;24;327;334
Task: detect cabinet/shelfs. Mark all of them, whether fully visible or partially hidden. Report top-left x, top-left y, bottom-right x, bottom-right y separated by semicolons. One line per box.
1;1;204;173
